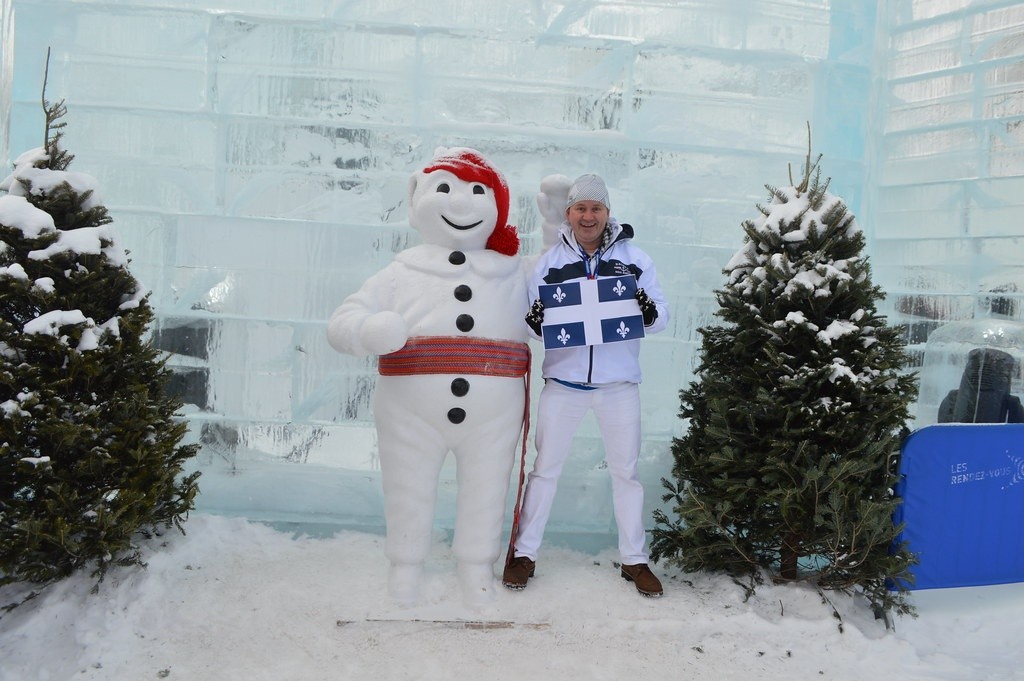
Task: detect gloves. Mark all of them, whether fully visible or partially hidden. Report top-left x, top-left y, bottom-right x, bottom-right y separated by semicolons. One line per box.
635;288;658;328
524;299;545;337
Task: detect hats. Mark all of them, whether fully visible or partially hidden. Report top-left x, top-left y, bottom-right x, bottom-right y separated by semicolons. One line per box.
566;174;610;211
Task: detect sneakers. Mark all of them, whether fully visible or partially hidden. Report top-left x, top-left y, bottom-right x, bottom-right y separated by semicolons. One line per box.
621;563;663;597
503;556;535;590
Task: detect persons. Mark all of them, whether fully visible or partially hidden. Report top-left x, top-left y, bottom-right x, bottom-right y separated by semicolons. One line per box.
326;147;576;604
502;174;664;598
